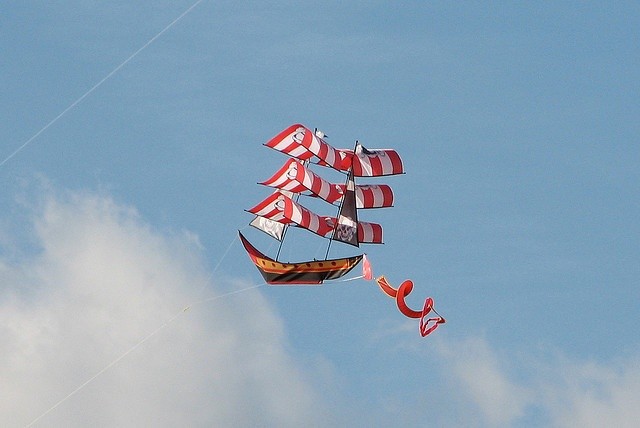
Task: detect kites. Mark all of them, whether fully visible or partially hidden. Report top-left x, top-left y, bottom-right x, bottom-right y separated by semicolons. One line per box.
238;123;445;337
